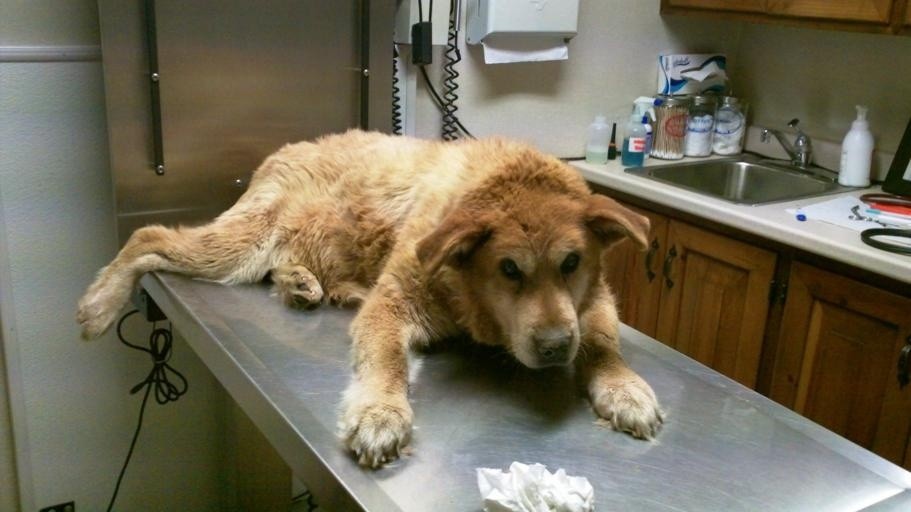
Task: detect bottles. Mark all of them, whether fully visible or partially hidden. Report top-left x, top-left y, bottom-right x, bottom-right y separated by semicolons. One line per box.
683;94;717;157
622;104;647;166
650;94;689;159
711;96;747;156
585;116;611;164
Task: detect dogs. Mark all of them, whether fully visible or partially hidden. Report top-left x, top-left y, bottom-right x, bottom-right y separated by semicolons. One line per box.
75;126;669;473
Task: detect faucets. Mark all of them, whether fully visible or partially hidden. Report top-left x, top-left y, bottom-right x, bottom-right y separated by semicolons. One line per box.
761;117;812;169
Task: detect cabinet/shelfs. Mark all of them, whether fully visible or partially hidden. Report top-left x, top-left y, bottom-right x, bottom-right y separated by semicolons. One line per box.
601;197;780;394
660;0;911;37
768;259;910;471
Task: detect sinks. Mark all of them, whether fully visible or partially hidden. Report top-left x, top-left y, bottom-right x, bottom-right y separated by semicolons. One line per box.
625;154;873;206
